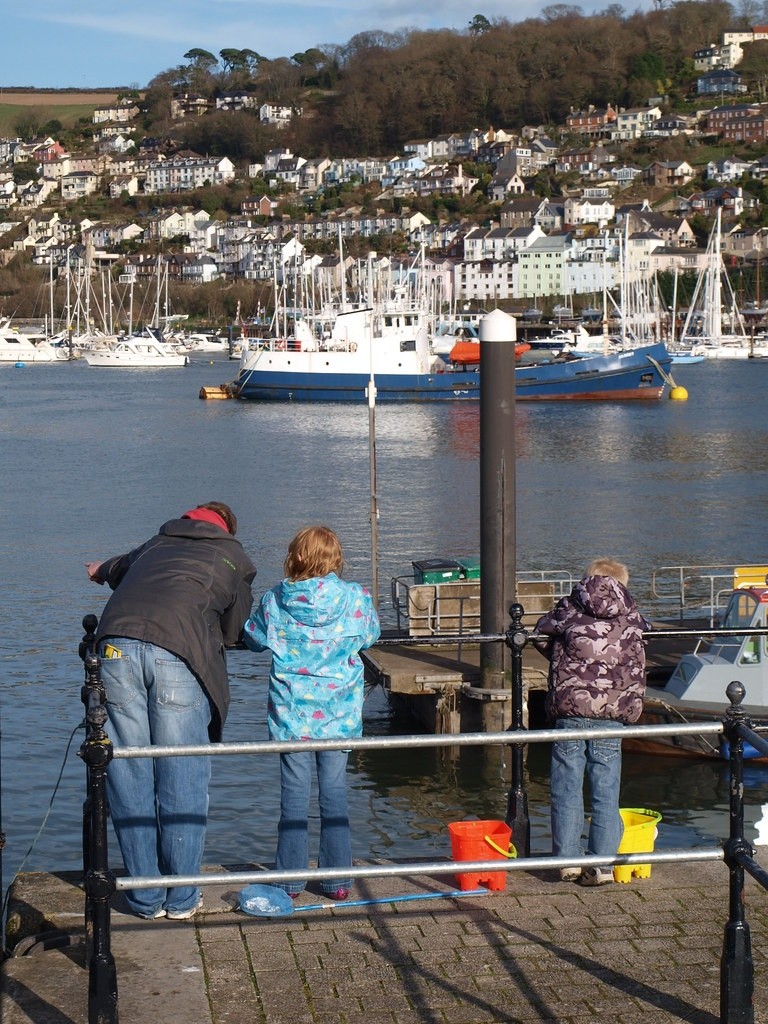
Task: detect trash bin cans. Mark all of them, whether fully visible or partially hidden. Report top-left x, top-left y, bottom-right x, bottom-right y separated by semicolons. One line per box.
411;559;461;583
452;556;481;578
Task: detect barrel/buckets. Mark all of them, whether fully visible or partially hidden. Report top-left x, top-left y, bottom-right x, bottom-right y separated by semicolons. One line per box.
588;809;662;882
447;820;519;891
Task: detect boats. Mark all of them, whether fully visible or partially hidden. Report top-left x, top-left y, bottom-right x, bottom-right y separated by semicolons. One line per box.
621;565;768;766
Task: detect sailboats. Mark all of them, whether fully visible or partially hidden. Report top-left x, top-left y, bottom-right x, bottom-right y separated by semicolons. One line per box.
232;224;674;404
0;206;768;368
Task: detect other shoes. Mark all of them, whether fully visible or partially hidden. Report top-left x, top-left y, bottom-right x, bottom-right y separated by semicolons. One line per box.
286;892;300;898
154;909;166;919
323;888;348;899
167;891;203;919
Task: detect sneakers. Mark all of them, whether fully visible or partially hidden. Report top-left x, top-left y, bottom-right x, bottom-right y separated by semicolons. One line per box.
559;868;581;882
574;867;614;885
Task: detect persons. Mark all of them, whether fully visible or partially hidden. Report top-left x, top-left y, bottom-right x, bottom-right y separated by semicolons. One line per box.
84;501;256;918
244;525;381;901
531;559;653;886
